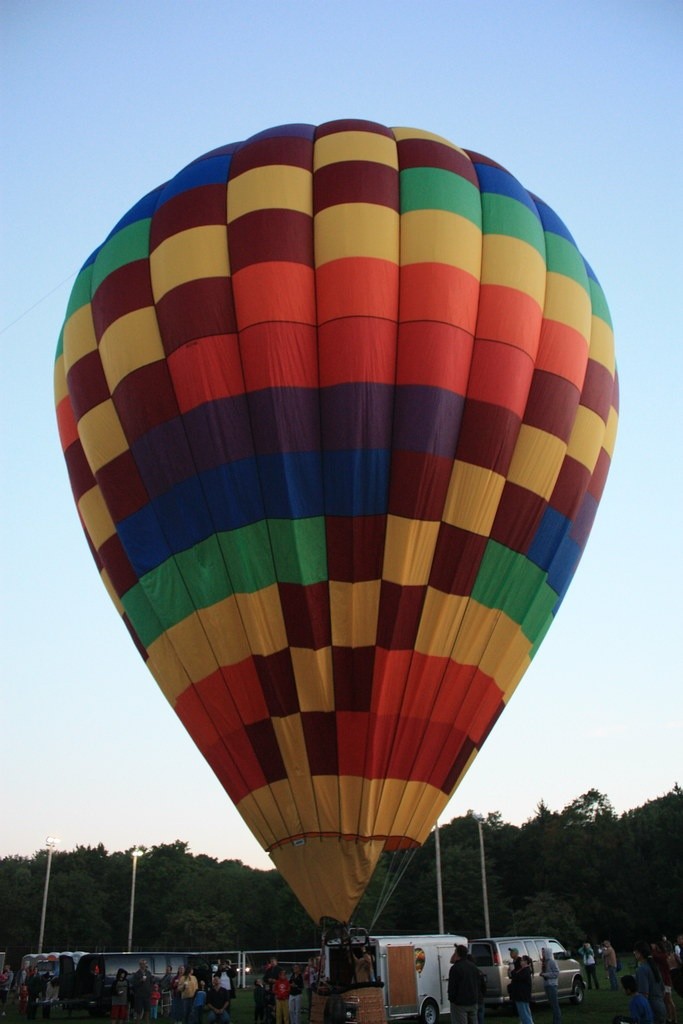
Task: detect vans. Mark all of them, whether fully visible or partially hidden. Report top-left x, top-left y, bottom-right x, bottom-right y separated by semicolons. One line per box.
468;936;587;1018
74;951;191;1019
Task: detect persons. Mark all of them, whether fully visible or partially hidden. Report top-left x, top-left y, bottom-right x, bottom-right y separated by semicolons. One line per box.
0;960;59;1018
621;935;683;1024
111;959;238;1024
507;948;534;1024
448;944;486;1024
540;947;561;1024
254;957;321;1024
351;945;376;984
578;940;617;991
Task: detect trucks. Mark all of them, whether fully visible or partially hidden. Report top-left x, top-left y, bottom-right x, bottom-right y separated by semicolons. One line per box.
323;933;467;1024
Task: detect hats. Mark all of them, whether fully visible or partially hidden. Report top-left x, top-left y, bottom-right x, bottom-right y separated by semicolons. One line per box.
454;944;468;958
508;948;518;953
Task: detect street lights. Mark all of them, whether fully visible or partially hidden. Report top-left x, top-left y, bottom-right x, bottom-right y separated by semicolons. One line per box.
50;121;619;1024
38;837;61;954
128;850;145;952
